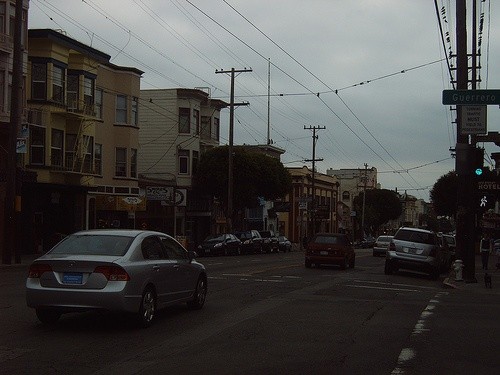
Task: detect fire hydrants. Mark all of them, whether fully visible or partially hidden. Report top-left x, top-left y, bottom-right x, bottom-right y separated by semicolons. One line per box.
451;259;465;281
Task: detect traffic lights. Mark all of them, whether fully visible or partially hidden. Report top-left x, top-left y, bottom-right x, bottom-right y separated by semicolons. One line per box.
471;148;484;179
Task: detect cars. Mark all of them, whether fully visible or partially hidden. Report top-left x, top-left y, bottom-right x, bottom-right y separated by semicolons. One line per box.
277;235;292;252
372;235;394;256
258;230;280;253
304;233;356;271
234;230;263;253
494;239;500;248
196;233;242;257
23;230;208;328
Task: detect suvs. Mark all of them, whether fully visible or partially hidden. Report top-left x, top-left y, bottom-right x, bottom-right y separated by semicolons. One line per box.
385;224;456;280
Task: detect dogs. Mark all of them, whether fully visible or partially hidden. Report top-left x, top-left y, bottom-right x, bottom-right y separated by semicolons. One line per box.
484;272;492;289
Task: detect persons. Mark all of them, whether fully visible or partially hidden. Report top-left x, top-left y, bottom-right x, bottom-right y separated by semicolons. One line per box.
480;231;492;270
